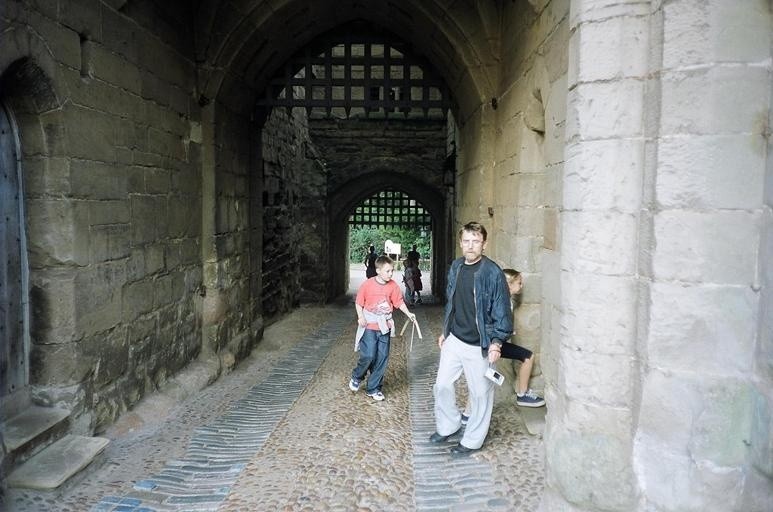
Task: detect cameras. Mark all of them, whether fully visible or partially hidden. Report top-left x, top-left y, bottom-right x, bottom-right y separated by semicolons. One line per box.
484;368;506;385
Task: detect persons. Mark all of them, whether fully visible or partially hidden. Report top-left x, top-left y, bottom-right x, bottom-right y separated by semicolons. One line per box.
501;268;546;407
349;256;415;402
365;246;379;278
403;245;423;307
429;221;514;456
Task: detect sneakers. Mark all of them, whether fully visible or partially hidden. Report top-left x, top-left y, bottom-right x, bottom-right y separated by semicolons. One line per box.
516;388;545;407
365;390;385;401
349;378;360;392
450;443;483;458
429;426;462;444
461;413;470;425
